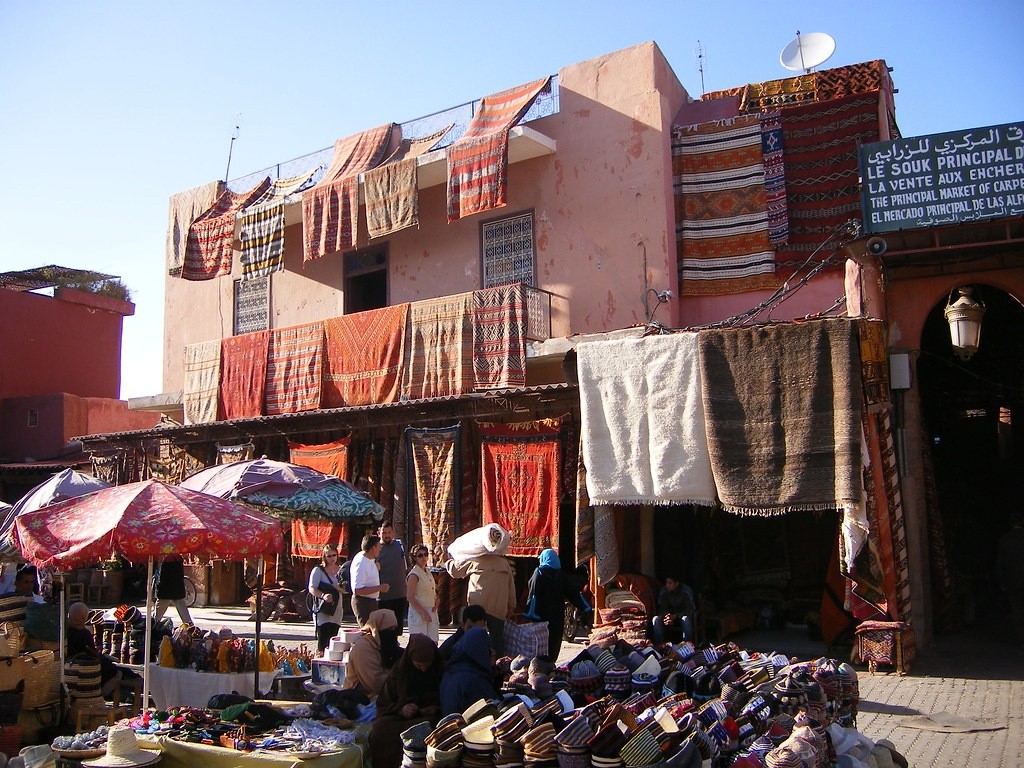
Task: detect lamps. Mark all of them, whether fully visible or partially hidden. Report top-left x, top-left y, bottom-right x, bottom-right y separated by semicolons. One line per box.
944;266;987;361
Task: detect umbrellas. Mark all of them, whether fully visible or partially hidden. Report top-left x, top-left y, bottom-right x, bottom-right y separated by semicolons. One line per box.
11;477;285;716
180;455;385;700
0;469;115;681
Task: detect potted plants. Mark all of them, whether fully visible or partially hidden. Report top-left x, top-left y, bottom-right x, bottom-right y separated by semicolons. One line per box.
802;610;822;641
102;551;129;603
89;564;103;595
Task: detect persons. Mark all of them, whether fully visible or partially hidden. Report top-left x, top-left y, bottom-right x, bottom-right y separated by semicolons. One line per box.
27;581;144;704
374;520;407;648
652;576;693;641
343;605;501;768
524;548;565;667
995;512;1024;647
407;545;439;647
308;545;343;653
442;553;516;657
151;554;194;628
350;536;390;627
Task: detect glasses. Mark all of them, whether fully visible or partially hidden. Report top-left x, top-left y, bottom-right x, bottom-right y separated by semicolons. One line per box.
325;554;337;558
417;553;428;558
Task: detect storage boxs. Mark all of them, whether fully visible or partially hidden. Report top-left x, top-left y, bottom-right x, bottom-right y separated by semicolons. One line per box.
311;658;348;686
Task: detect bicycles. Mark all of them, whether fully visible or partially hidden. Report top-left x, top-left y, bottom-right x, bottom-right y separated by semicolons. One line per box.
151;568;196;608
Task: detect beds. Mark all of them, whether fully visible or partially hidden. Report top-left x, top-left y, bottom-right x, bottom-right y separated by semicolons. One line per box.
698;610;755;642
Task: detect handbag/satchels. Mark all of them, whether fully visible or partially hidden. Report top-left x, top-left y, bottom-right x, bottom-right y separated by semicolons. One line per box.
309;565;339;616
502;611;549;660
0;592;107;752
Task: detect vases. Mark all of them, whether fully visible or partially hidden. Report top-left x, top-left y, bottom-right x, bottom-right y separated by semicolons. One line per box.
76;568;91;594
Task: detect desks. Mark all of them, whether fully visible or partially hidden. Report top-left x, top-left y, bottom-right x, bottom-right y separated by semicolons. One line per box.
149;662;285;711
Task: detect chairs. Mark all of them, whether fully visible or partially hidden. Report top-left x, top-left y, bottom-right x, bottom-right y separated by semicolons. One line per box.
664;592;706;641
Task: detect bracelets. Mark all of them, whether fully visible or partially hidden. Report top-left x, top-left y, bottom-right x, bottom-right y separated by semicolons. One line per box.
366;587;371;595
320;593;325;599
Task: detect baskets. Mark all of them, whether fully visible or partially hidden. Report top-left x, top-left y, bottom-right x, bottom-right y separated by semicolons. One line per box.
51;743;107;758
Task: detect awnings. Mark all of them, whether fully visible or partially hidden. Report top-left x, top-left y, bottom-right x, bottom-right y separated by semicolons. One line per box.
69;382;578;442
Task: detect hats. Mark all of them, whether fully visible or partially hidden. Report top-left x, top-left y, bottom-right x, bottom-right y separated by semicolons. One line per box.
80;726;162;768
398;640;908;768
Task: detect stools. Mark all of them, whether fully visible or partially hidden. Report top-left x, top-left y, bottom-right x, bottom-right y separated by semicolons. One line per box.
114;679;144;716
87;585;111;604
76;707;126;734
65;579;84;605
